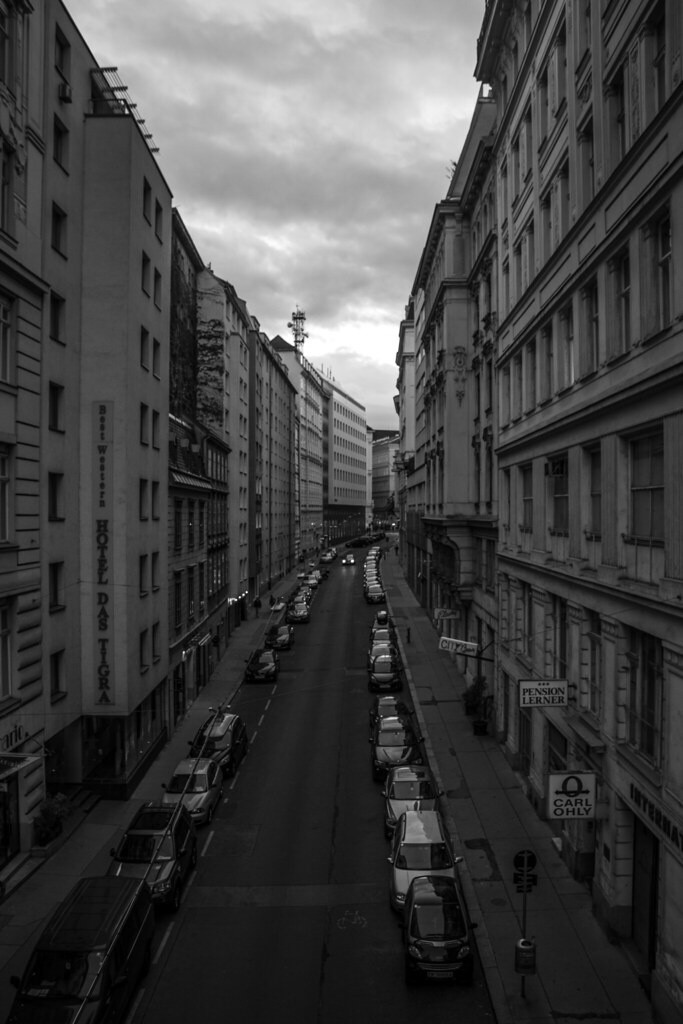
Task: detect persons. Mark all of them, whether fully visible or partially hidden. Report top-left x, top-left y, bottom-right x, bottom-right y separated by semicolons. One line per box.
308;559;314;567
269;595;276;606
394;544;399;555
300;554;304;562
254;596;262;616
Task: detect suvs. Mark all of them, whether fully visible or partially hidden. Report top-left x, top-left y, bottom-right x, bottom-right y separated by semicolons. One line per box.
107;799;199;916
5;874;159;1024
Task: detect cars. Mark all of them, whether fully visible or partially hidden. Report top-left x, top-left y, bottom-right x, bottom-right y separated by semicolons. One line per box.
187;712;249;778
264;624;296;649
283;531;427;783
386;809;464;910
397;874;478;983
380;763;445;840
243;647;281;684
160;757;225;826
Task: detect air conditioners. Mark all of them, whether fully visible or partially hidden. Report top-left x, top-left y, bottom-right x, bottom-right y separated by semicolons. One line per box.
60;83;72;103
